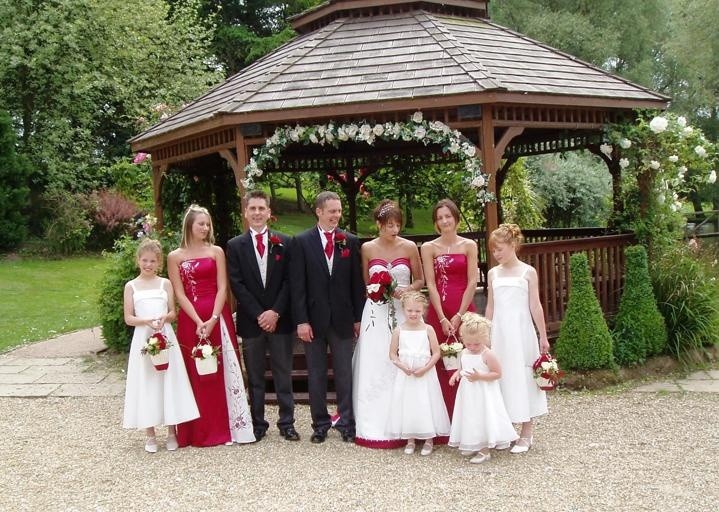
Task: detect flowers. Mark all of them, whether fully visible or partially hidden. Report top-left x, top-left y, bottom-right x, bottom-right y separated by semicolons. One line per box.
334;231;351;258
438;339;464;356
140;332;174;357
321;172;370;198
275;254;280;261
190;342;222;359
593;105;719;230
237;110;498;207
365;272;399;307
533;354;565;389
268;236;284;253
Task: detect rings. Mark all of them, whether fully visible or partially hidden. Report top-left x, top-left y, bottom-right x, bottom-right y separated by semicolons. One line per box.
267;324;270;327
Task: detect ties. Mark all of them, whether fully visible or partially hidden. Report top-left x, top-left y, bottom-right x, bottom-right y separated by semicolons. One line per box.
322;232;333;260
255;234;265;259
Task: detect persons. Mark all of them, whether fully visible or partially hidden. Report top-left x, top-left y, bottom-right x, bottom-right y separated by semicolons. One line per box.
225;189;300;440
167;203;257;447
448;312;520;464
286;191;366;443
123;238;200;453
330;198;425;449
389;290;451;456
483;223;550;454
421;198;477;445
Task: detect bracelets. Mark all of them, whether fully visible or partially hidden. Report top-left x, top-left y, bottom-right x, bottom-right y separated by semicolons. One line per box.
437;317;448;323
211;315;220;321
457;312;463;319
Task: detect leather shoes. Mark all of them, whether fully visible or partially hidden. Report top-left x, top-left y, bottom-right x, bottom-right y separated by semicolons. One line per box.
340;429;356;442
470;451;491;464
510;435;533;454
145;434;158;453
310;429;327;443
166;432;179;450
421;440;433;456
280;427;299;440
249;428;266;442
404;443;416;454
